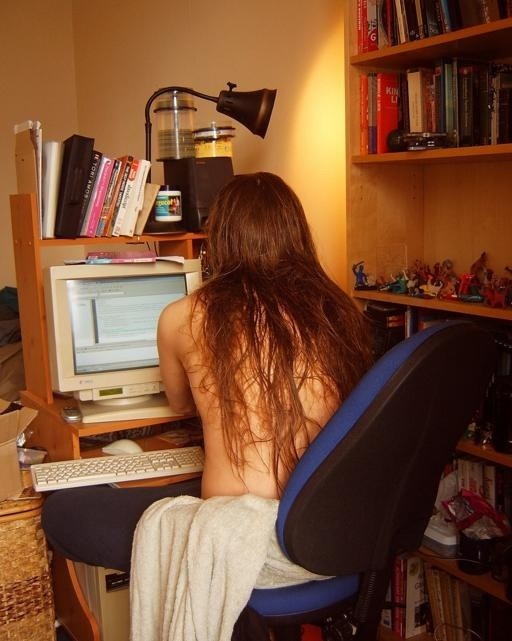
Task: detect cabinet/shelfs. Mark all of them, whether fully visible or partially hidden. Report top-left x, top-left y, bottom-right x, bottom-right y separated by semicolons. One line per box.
345;0;512;640
7;192;216;640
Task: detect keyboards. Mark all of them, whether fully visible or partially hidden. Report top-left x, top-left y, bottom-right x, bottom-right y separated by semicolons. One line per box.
29;445;205;492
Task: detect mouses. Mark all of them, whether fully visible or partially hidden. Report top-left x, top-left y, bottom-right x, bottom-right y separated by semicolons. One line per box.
102;438;144;455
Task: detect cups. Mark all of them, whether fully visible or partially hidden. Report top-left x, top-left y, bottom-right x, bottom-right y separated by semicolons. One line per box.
458;528;497;575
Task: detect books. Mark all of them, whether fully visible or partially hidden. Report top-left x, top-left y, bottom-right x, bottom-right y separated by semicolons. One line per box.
85;251;156;264
357;1;512;55
42;134;153;238
359;57;512;154
382;550;484;641
456;455;508;514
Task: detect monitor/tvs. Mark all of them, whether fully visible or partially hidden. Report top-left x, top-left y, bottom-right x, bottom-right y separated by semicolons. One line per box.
43;260;202;423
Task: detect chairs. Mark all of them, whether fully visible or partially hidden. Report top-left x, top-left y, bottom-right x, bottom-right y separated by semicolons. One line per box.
240;320;498;641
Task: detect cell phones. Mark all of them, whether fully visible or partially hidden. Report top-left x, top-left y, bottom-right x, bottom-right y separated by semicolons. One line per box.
61;402;83;423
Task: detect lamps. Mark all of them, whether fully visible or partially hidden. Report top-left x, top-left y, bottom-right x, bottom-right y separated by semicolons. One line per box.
144;81;278;179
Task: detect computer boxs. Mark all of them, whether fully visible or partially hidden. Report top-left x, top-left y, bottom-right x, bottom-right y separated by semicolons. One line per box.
71;560;131;641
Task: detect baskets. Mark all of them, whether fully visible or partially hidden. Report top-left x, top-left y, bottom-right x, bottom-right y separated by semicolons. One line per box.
0;508;58;641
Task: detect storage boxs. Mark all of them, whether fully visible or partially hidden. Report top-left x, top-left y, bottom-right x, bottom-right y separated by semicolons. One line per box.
0;399;37;504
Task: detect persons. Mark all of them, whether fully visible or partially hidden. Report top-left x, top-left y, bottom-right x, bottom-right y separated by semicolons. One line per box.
157;171;379;590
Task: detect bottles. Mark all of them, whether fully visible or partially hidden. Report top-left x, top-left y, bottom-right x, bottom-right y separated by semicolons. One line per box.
154;185;183;222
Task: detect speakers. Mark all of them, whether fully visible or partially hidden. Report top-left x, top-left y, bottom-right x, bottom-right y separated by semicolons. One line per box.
163;155;235;232
